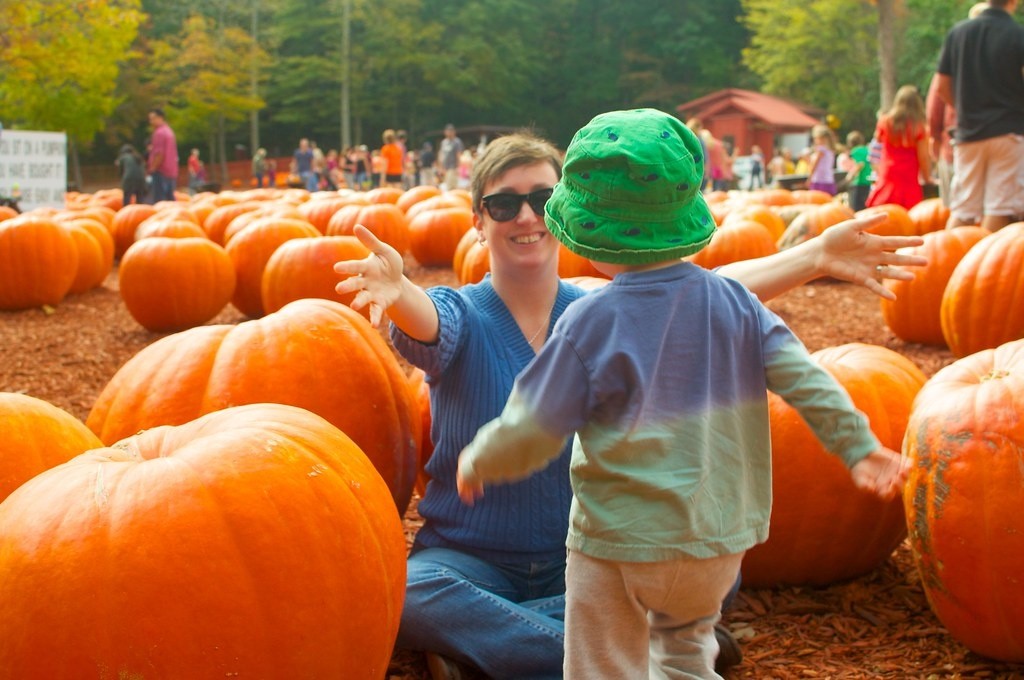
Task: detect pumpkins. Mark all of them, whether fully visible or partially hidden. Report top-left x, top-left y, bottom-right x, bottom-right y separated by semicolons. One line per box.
0;171;1024;680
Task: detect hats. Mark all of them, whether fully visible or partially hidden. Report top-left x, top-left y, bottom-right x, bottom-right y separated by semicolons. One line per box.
543;108;721;265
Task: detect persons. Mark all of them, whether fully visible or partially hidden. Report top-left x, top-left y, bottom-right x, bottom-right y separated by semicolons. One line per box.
691;0;1024;212
454;107;910;680
331;132;928;680
118;108;475;210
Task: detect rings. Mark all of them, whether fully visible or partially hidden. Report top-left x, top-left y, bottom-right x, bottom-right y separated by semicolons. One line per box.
876;265;884;278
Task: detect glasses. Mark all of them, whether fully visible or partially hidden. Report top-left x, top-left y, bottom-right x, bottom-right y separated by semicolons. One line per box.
482;187;553;222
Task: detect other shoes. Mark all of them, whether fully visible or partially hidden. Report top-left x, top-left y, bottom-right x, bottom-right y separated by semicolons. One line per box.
714;624;742;668
